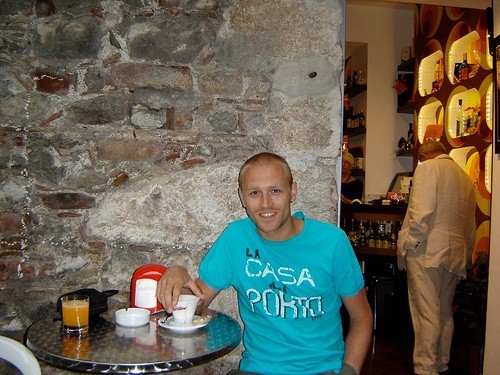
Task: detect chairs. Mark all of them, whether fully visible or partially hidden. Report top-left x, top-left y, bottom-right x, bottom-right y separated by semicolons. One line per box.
338;279;378;343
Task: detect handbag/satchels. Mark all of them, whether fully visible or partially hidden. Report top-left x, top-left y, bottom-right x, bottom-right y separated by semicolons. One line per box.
55;287;120;321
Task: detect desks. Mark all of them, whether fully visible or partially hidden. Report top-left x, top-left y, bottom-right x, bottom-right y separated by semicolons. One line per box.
25;302;241;375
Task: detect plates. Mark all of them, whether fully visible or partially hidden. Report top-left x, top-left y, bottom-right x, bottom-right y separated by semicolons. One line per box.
157;315;209;333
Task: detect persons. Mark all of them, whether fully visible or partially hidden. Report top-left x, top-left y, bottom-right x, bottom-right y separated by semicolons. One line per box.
397;140;476;375
340;151;364;232
156;152;373;375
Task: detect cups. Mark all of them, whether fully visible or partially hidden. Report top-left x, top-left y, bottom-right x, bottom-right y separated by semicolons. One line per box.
61;294;89;334
171;293;200;323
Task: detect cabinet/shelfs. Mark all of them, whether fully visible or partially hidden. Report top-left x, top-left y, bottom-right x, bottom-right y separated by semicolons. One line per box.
341;203;411;334
395;56;413;158
343;82;364;176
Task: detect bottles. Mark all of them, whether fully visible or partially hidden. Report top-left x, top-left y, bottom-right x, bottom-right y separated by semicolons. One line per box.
459;52;469;80
456;99;464;137
341;217;403;250
343;106;365;131
345;69;367;94
408;123;414;150
